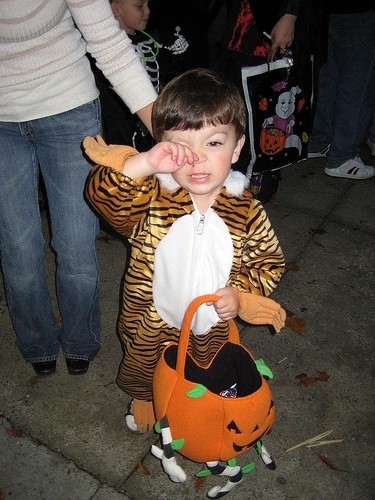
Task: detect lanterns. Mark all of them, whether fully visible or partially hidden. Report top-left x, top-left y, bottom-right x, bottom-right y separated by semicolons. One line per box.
149;295;278;499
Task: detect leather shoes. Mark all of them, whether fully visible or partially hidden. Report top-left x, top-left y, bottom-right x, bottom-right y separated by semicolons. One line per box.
64;358;90;374
33;360;56;375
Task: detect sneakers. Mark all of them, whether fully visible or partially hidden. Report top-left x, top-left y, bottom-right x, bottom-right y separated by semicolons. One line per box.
308;144;331;158
323;153;375;179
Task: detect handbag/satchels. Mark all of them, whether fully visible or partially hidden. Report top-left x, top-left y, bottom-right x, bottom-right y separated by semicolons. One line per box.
150;295;278;497
241;44;314;178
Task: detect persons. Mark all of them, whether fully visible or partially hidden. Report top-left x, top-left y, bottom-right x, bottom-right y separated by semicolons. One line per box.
81;67;284;436
0;1;162;377
296;0;375;181
81;1;186;239
152;1;304;209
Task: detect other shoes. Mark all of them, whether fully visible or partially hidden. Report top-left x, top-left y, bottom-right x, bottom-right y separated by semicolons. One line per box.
124;399;149;433
249;170;280;205
